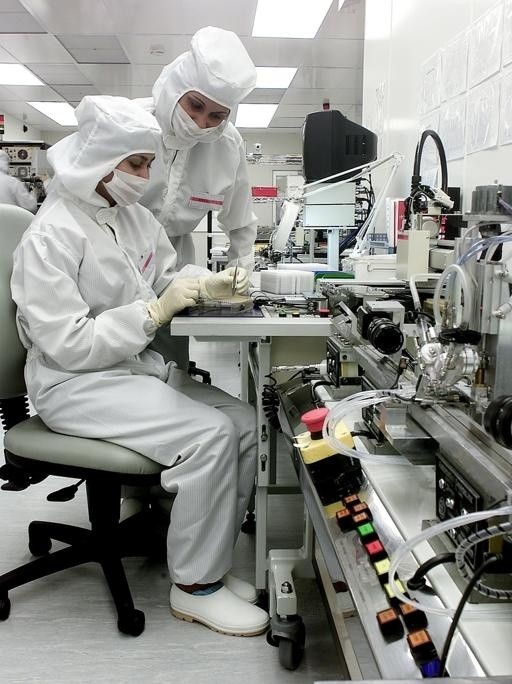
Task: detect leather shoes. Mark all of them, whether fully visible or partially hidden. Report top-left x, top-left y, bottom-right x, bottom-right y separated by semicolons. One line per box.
221;574;259;605
168;585;270;636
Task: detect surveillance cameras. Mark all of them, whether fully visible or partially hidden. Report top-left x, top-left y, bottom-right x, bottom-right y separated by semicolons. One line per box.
254;143;262;150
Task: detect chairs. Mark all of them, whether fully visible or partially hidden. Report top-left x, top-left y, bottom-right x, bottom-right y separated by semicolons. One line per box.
1;202;174;636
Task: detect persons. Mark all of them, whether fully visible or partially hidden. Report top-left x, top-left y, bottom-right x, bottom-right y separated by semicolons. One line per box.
7;93;271;636
117;26;257;525
0;150;37;215
39;173;53;197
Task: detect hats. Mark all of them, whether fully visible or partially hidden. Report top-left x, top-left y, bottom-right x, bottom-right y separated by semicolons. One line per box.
46;95;163;227
151;27;257;151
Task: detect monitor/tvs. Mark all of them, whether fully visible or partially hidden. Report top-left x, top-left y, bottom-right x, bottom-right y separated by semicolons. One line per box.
303;110;379;184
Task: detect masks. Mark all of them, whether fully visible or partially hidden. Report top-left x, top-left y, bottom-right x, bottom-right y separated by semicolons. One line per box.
168;103;224;143
101;169;149;207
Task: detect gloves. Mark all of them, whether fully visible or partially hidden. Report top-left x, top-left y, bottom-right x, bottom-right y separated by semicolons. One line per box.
147;277;201;327
203;267;254;302
